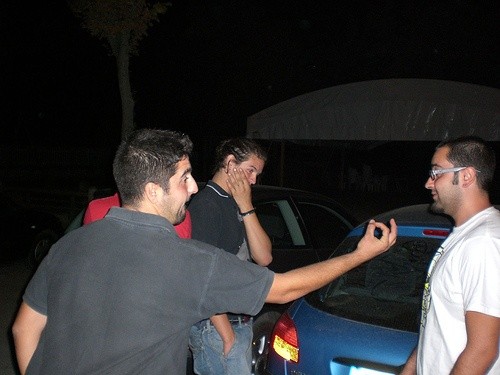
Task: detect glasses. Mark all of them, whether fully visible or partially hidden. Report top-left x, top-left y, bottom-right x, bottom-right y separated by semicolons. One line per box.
429;167;482;181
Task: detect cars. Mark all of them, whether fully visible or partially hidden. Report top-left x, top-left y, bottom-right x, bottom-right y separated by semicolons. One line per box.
265;206;454;375
243;183;367;374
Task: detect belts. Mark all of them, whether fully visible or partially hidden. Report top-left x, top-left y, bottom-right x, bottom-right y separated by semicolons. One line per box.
231;315;250;325
